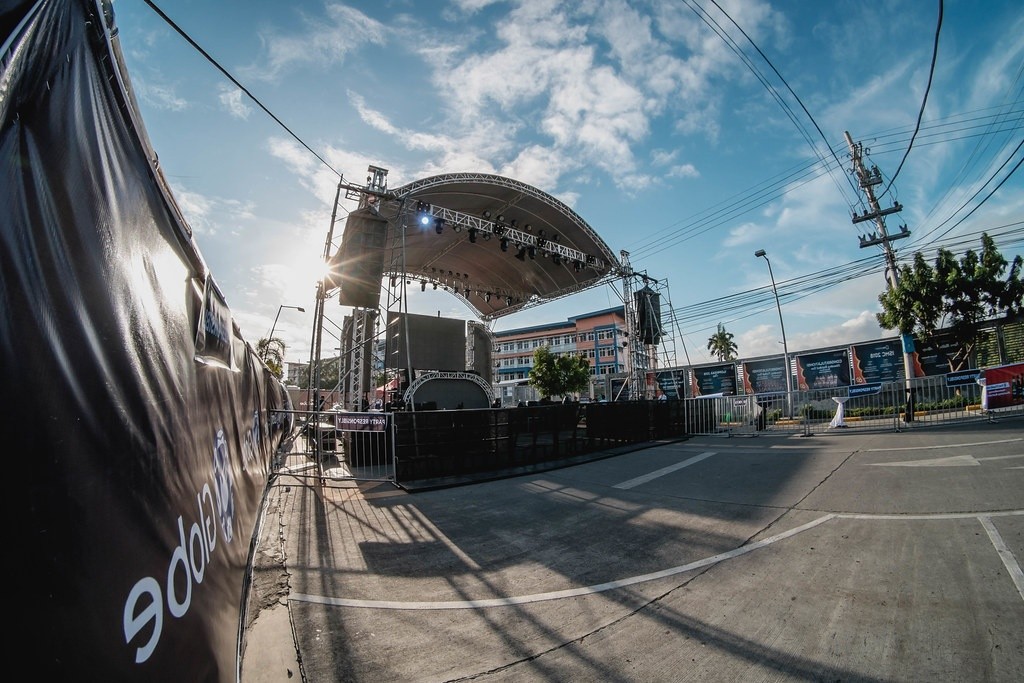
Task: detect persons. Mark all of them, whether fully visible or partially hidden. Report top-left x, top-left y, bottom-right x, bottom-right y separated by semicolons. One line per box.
656;387;666;403
538;394;607;405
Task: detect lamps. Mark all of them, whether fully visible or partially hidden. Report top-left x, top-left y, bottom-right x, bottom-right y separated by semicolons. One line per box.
491;397;501;408
391;276;397;287
385;398;406;412
406;266;513;308
417;200;595;273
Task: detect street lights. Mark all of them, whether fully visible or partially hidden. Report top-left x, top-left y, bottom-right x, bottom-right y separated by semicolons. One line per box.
755;248;794;420
265;305;305;362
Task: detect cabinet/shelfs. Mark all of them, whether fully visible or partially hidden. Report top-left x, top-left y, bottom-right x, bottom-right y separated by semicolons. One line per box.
298;402;313;434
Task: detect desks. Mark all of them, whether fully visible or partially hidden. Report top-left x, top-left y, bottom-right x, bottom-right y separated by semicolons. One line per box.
307;421;338;459
